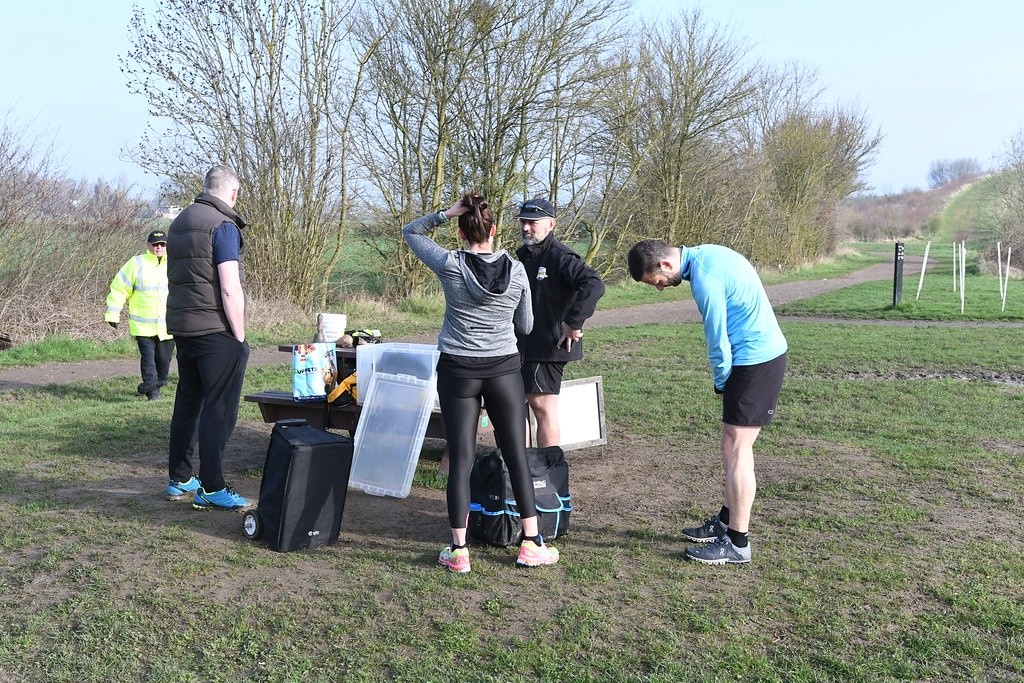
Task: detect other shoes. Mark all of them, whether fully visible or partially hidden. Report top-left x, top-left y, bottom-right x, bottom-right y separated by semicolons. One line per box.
144;387;161;400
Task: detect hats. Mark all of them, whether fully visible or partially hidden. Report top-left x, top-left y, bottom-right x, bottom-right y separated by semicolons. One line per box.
513;199;555;221
148;230;167;244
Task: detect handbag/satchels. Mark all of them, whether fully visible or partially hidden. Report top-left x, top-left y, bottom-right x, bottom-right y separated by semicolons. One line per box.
462;445;571;549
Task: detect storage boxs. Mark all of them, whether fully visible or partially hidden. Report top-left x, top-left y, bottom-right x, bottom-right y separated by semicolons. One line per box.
356;342;485;414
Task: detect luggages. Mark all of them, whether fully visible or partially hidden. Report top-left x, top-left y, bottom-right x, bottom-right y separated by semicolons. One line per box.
242;419;354;554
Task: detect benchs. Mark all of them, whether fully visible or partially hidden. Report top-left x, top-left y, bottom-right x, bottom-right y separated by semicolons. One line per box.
243;343;495;479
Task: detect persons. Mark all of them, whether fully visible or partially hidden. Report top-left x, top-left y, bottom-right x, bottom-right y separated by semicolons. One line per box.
105;230;176;402
401;188;560;577
165;164;257;511
513;200;606;449
629;239;788;567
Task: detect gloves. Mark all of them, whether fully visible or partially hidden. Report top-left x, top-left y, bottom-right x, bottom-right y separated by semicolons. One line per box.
108;321;119;330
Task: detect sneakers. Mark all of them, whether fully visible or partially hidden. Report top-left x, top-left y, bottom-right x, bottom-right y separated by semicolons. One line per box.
681;513;728;543
164;474;201;501
682;532;752;563
515;534;559;567
191;483;259;513
439;546;471;572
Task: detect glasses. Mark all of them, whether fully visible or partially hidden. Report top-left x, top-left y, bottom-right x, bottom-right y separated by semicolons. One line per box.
152;242;166;247
518;202;554;218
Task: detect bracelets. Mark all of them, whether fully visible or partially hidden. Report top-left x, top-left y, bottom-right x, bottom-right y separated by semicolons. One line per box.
438;209;450;223
